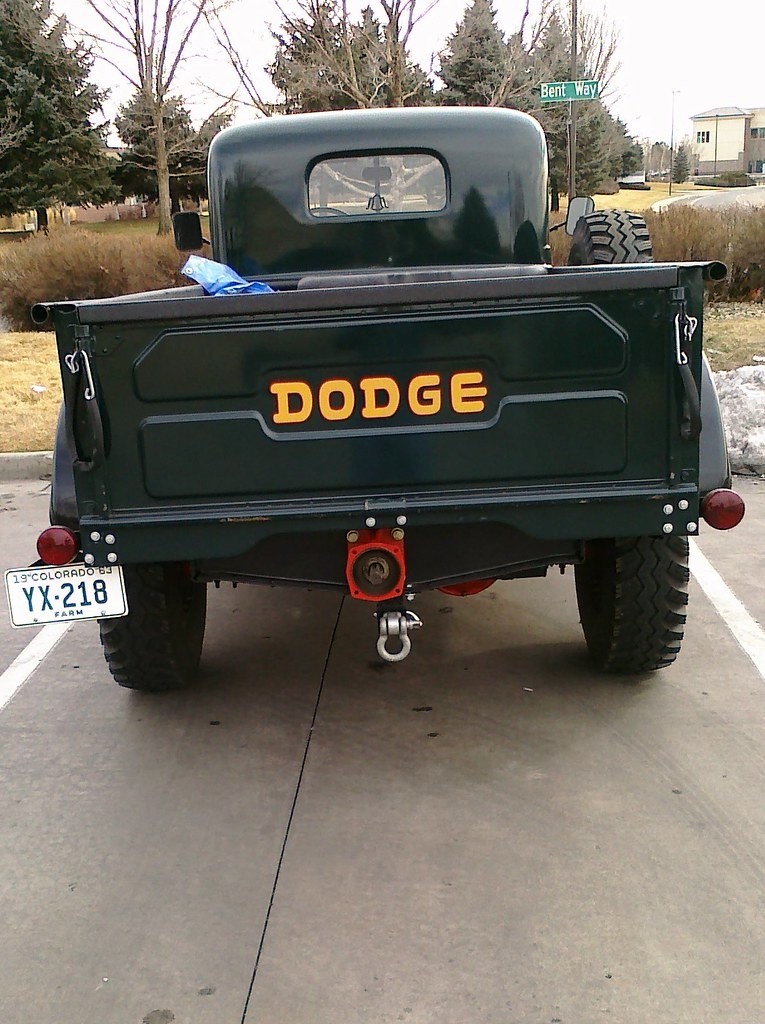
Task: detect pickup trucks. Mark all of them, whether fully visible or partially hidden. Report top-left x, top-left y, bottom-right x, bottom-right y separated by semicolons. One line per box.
3;106;747;700
